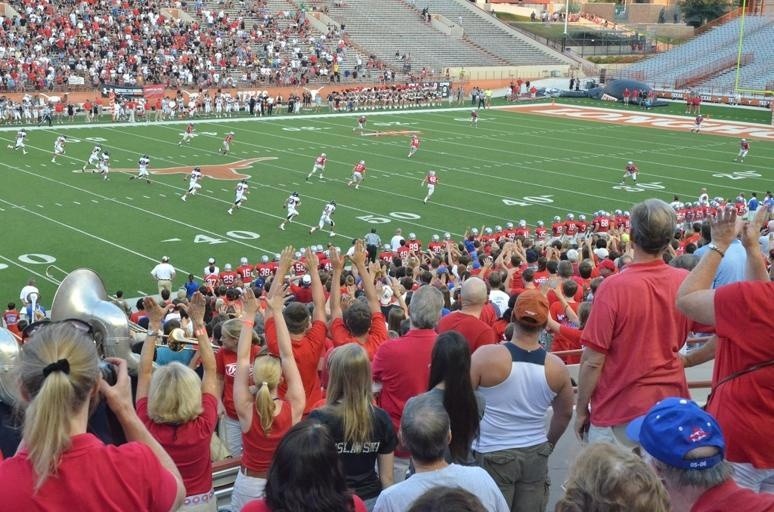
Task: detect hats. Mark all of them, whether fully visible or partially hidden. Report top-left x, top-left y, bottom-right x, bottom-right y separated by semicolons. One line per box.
513;289;549;328
381;285;393;306
162;255;171;261
303;274;312;284
625;397;724;470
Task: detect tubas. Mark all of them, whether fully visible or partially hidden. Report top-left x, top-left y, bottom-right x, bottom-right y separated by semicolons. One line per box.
50;267;158;374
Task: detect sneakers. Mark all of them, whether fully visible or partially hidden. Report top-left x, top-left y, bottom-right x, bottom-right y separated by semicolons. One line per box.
330;232;335;236
279;226;285;231
227;210;232;216
181;197;186;202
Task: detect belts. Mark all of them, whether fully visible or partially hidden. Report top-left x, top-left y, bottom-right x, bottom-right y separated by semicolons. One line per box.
241;465;268;479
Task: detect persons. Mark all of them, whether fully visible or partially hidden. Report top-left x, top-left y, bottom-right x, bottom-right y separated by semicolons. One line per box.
7;122;250;215
619;160;640;186
0;1;537;128
306;115;439;205
623;87;656;110
684;88;751;164
1;187;772;511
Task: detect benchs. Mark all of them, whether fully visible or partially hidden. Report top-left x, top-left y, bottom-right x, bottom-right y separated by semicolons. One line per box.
607;9;774;101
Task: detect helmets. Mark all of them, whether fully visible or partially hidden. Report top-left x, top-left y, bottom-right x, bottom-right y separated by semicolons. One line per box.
432;234;439;242
225;264;232;272
260;245;340;263
293;191;299;197
408;233;417;242
241;179;247;184
537;209;630;227
208;258;215;263
330;200;336;206
384;243;390;252
195;167;199;172
673;197;724;210
472;219;527;234
240;256;247;266
443;232;451;240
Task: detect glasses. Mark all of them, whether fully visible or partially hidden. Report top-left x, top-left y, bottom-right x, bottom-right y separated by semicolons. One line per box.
22;319;95;345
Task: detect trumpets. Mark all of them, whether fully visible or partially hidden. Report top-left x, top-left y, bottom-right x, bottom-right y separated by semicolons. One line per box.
156;327;221;352
